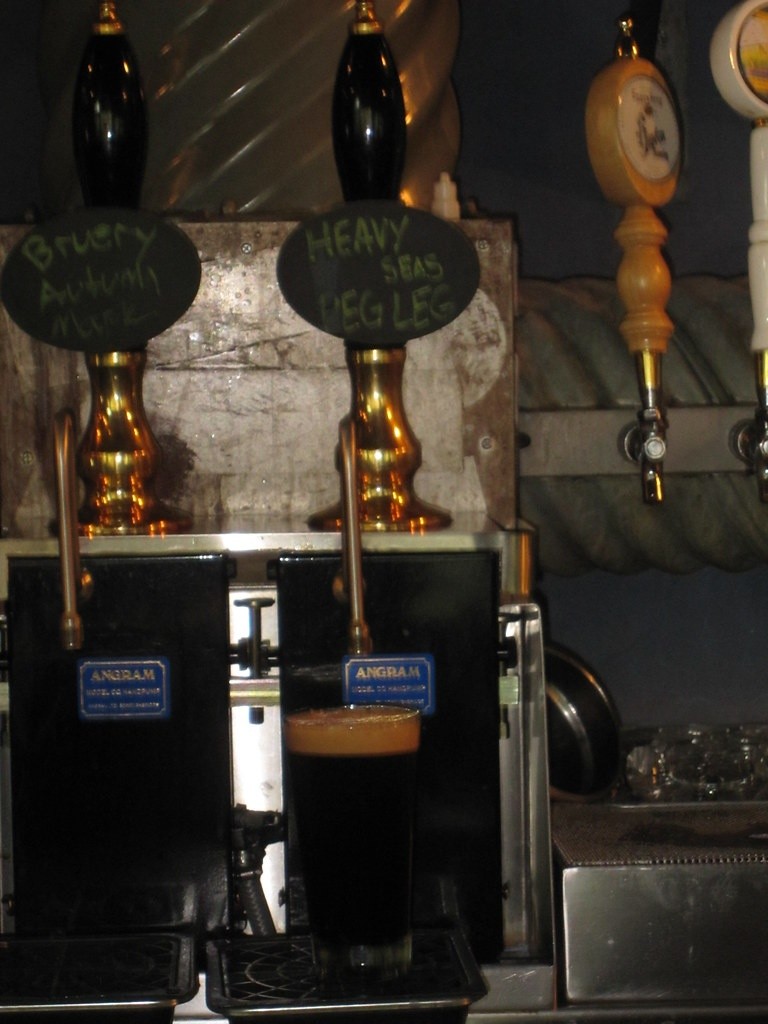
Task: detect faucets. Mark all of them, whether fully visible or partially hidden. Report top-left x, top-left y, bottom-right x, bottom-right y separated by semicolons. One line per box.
52;409;95;652
337;417;369;658
